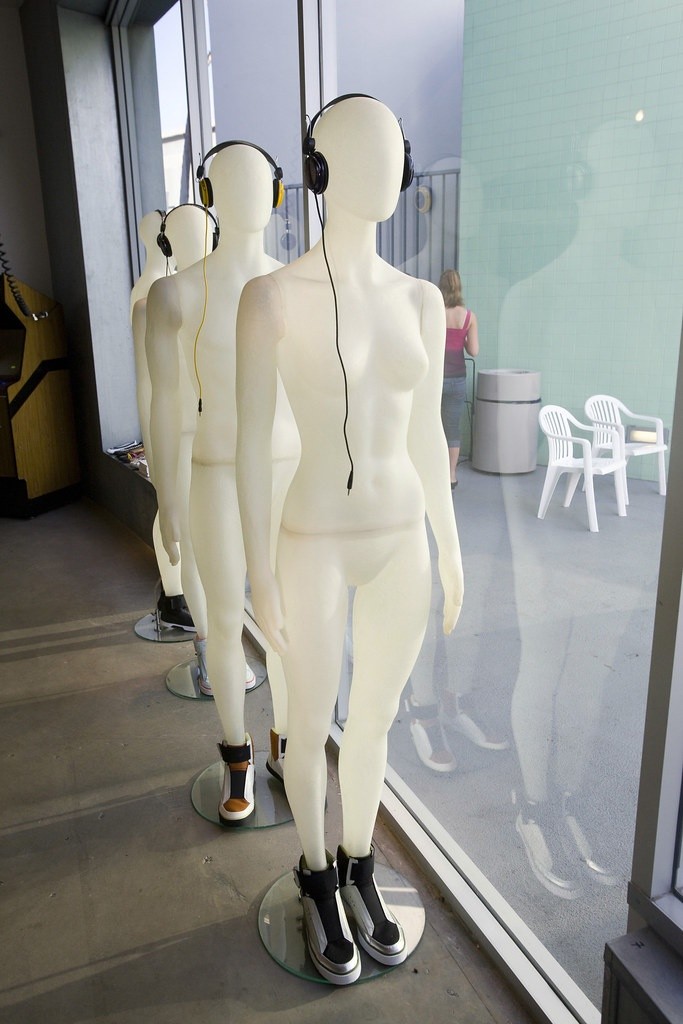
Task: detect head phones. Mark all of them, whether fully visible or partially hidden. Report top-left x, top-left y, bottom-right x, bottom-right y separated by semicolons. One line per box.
302;93;415;195
195;140;285;209
158;203;220;257
155;209;167;247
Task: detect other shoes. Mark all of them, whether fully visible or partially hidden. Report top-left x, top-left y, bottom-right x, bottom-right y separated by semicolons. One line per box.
451;480;459;490
107;440;146;469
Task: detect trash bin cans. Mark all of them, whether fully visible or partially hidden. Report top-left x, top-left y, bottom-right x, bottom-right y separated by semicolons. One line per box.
470;368;542;474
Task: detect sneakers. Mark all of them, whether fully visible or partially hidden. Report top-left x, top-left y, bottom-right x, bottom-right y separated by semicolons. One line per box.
556;788;624;886
159;595;197;632
337;842;408;966
217;734;256;828
439;687;512;752
157;581;165;612
515;794;583;898
404;695;460;778
292;848;362;985
244;661;256;690
266;728;287;784
194;635;213;696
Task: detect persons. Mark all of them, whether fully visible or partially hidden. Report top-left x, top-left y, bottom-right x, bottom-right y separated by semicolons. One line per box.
131;207;197;631
235;91;467;987
153;202;255;697
143;141;329;820
434;269;478;486
407;581;510;771
490;131;643;886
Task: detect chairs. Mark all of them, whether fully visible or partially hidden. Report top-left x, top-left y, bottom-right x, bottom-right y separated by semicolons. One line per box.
581;394;667;505
538;405;627;532
